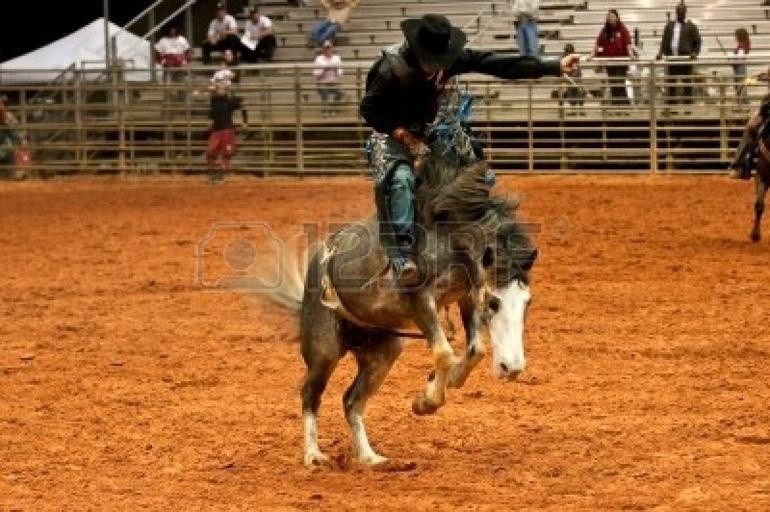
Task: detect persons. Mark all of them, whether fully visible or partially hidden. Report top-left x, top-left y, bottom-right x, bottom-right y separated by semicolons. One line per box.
656;4;701;104
152;28;194;83
358;14;579;286
510;1;541;61
728;29;750;104
211;63;235;89
307;0;360;47
242;7;276;64
589;11;637;104
224;49;240;88
314;40;345;117
561;43;580;84
207;82;247;175
202;3;237;64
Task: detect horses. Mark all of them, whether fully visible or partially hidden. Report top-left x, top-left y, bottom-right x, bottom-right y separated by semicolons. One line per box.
750;94;770;243
252;87;539;474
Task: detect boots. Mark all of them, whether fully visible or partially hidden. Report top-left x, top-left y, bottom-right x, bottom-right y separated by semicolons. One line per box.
395;233;417;279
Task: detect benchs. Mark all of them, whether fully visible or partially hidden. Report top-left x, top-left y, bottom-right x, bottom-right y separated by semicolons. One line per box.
94;2;770;121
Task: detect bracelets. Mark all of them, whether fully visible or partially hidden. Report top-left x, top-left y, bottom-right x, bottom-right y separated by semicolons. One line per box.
399;131;409;142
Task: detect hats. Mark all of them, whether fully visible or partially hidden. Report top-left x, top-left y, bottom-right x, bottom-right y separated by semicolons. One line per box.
399;13;469;64
